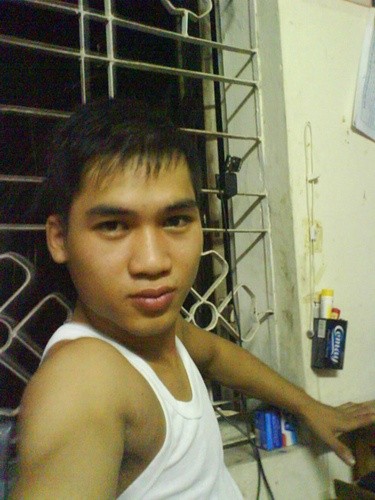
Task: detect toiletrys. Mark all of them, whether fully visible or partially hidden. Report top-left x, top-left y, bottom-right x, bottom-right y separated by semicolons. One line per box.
311;289;349;319
252;405;299;452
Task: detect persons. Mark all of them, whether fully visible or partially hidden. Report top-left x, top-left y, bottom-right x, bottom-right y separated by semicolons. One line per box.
7;95;375;499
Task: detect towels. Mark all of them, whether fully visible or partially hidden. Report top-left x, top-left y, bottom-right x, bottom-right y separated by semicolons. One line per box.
350;0;375;142
